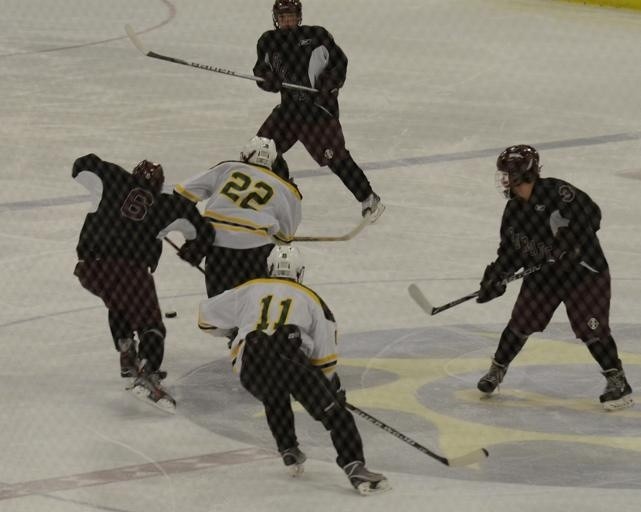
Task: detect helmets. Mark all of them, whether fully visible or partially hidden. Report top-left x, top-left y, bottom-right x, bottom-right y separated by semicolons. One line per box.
271;0;303;29
240;134;279;170
266;244;307;284
132;159;166;192
495;144;540;199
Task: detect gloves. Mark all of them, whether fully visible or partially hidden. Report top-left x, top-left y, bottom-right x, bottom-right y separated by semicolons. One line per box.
476;263;507;304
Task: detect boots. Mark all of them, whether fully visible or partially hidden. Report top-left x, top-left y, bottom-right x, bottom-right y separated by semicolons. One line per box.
476;359;510;394
599;359;633;404
133;366;181;409
279;447;312;467
360;190;381;220
115;339;138;377
342;459;388;487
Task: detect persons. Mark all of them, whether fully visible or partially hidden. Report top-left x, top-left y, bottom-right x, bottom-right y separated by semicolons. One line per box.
252;0;381;217
70;152;216;407
196;241;386;490
172;134;303;298
476;143;636;403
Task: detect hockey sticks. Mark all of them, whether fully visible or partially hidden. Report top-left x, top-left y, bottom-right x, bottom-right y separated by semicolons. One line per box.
293;215;371;241
346;403;488;466
409;264;541;315
125;23;338;93
